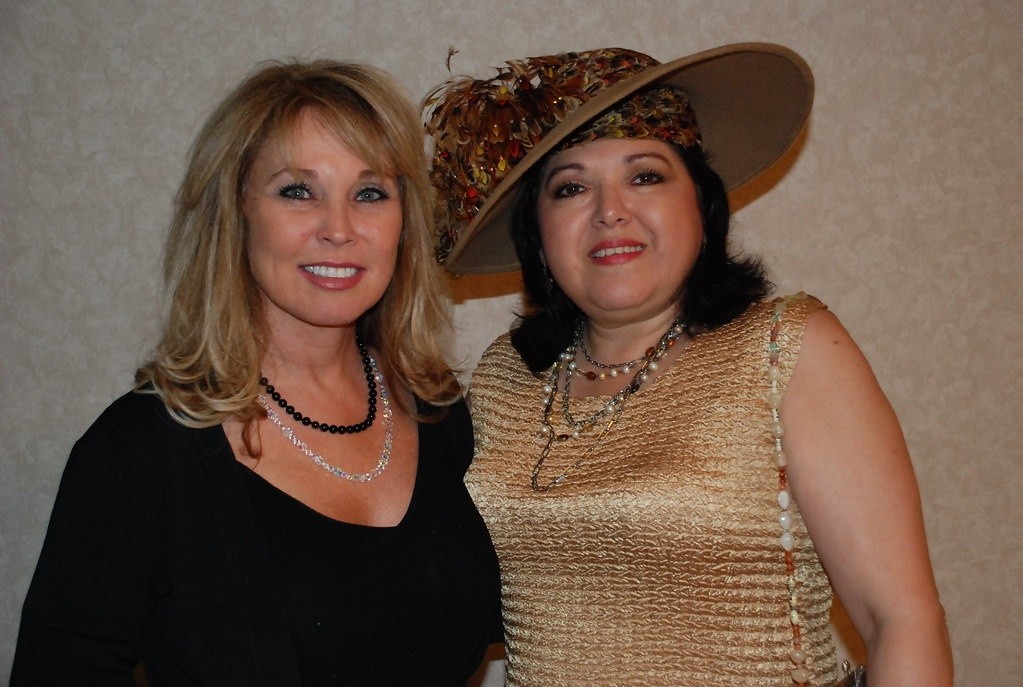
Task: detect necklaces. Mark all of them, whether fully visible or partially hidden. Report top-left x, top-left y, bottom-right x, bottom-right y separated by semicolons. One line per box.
259;334;377;434
530;317;688;493
258;353;395;483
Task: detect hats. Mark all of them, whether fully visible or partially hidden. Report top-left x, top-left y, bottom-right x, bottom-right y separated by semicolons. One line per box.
413;41;815;301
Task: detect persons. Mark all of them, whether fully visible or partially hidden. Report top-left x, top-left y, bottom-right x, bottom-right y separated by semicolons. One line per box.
7;56;509;687
426;41;955;687
